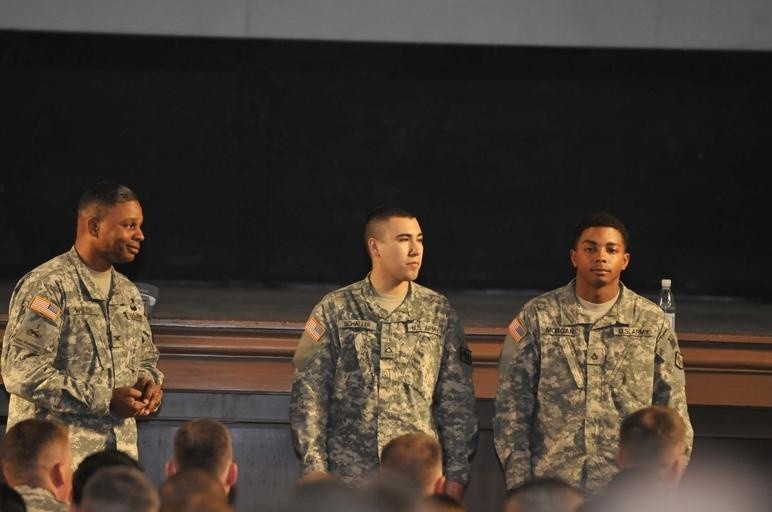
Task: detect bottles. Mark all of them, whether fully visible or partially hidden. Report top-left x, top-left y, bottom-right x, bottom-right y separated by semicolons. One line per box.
656;278;676;329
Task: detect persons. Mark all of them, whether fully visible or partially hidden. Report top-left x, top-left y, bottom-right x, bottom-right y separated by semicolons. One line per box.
490;212;694;498
1;176;164;493
289;207;479;502
